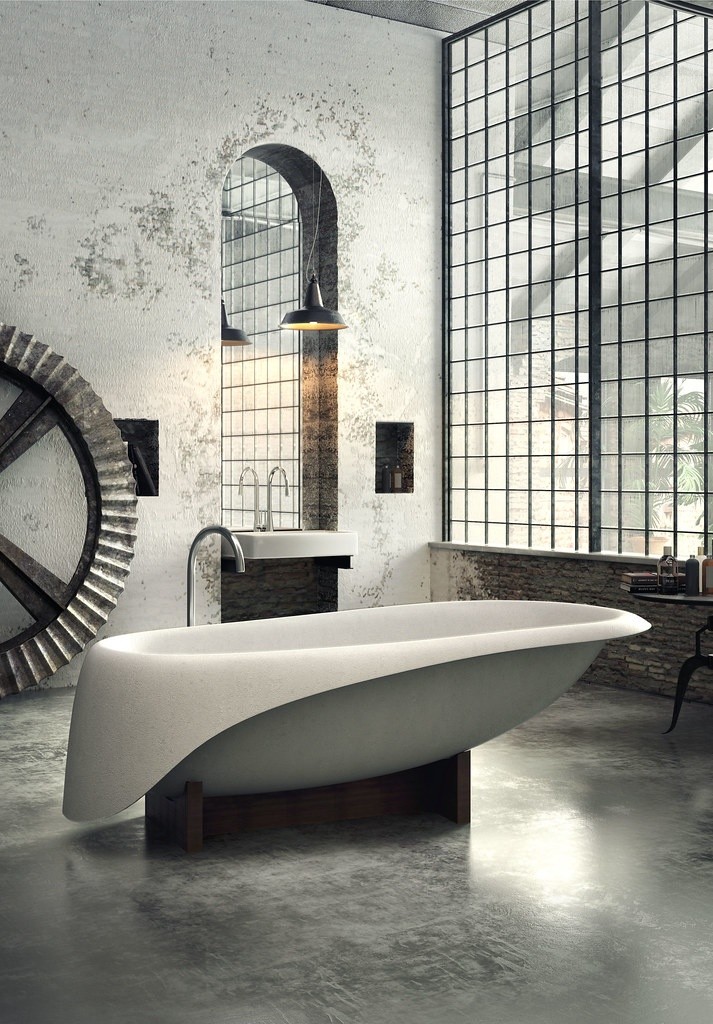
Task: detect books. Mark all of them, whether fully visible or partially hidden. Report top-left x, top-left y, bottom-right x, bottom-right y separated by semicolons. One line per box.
620;572;686;593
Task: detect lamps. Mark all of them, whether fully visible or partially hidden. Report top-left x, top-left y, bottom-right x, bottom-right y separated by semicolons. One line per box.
221;300;251;347
279;271;348;331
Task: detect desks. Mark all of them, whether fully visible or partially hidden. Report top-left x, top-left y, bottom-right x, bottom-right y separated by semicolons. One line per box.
633;592;713;735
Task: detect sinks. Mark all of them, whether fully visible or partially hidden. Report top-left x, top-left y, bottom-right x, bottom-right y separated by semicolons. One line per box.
220;530;358;560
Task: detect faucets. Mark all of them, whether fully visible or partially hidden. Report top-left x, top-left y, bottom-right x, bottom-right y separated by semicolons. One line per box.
265;466;290;532
238;467;263;532
186;523;246;628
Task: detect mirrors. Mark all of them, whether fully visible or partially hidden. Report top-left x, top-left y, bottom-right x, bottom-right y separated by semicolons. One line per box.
221;155;304;532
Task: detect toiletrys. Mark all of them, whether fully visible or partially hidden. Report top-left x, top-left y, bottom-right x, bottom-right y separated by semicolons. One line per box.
379;459;404;492
656;547;713;596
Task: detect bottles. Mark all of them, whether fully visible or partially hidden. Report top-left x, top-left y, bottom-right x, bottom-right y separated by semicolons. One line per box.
657;547;678;595
394;465;402;490
696;547;708;593
685;555;699;595
702;555;713;596
382;466;391;490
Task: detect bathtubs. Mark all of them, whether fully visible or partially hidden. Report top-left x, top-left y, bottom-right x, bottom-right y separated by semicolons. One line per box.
61;598;652;822
